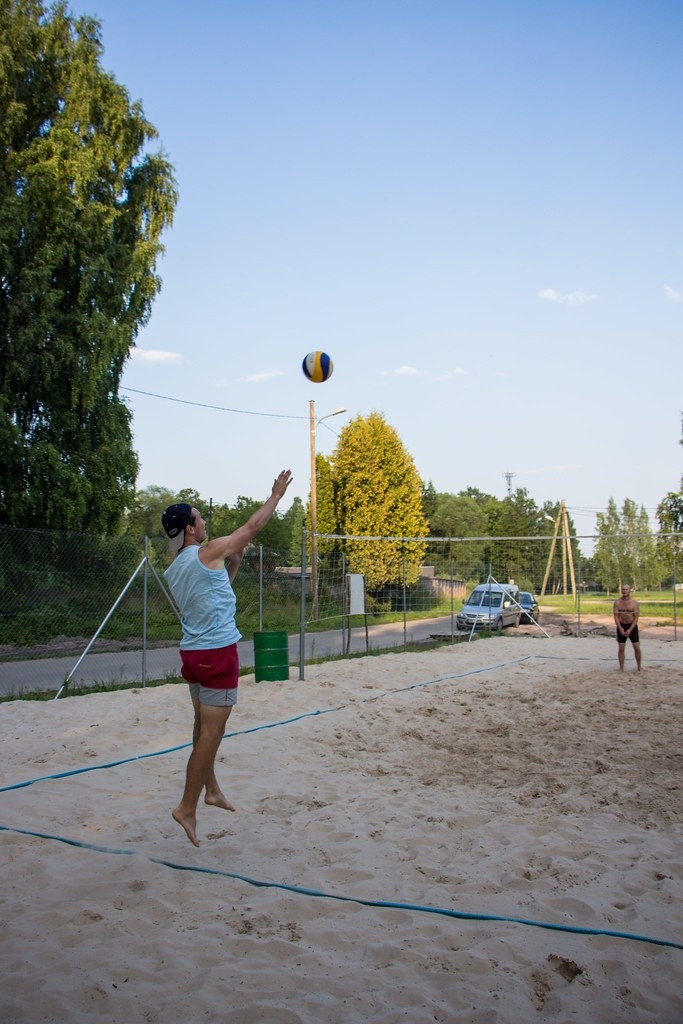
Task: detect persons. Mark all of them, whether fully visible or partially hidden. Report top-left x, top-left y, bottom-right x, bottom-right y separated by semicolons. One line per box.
161;470;293;848
613;585;647;672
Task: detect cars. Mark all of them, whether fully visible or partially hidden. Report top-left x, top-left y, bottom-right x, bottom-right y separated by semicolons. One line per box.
519;591;540;625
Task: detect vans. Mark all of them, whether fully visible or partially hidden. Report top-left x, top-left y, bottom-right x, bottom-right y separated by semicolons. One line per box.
456;583;522;633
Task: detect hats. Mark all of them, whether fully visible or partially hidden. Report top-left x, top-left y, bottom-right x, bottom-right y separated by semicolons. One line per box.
161;504;192;551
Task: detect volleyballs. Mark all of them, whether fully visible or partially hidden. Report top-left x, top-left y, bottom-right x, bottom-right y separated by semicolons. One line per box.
302;350;334;383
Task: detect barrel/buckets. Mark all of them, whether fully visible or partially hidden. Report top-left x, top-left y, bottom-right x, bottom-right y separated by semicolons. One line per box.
253;630;290;683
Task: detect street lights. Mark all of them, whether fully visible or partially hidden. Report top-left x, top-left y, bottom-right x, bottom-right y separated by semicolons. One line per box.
309;399;347;612
